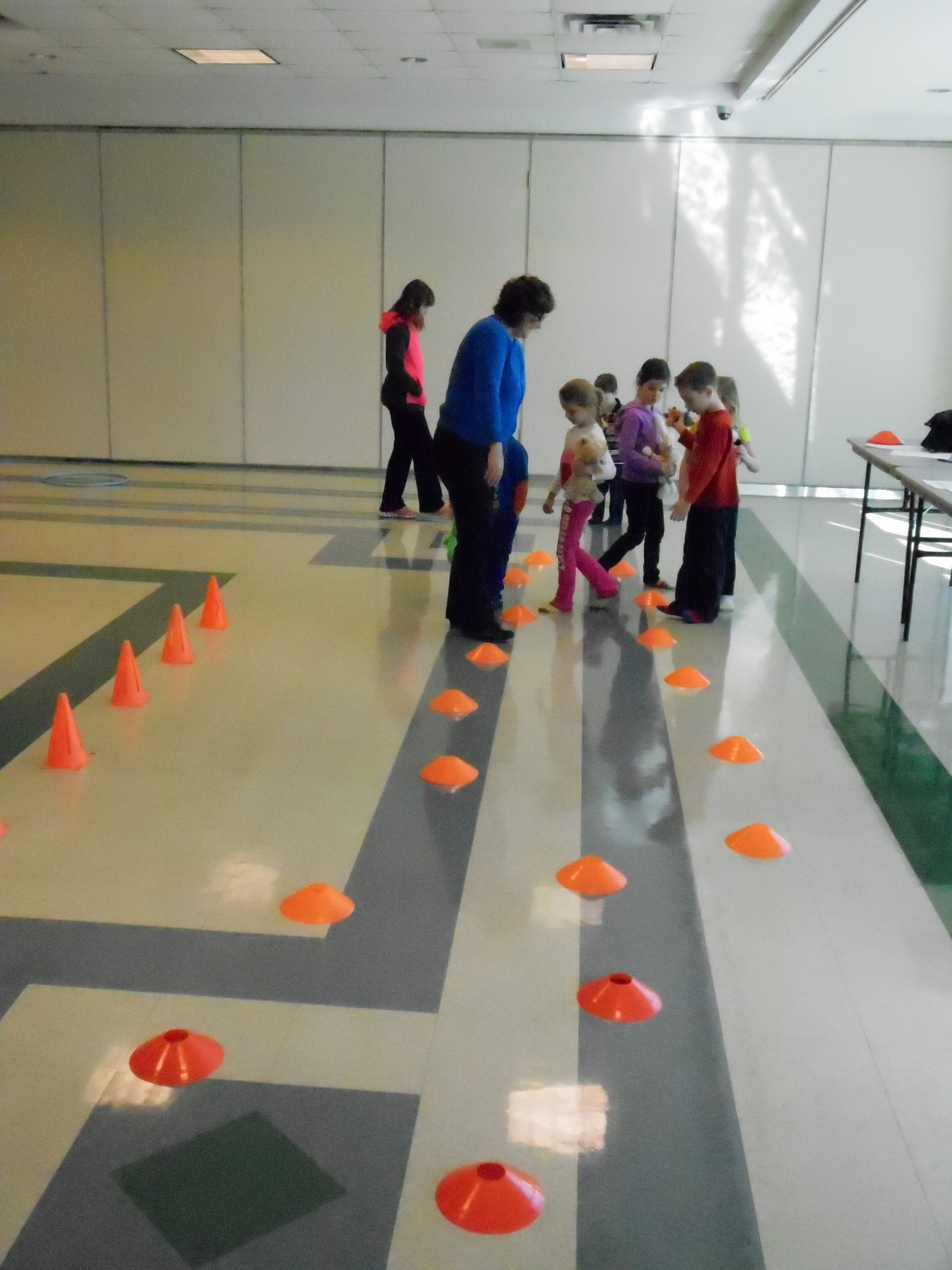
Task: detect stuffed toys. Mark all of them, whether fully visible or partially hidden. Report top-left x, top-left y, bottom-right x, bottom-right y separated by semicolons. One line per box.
564;435;608;504
665;406;695;427
643;434;678;499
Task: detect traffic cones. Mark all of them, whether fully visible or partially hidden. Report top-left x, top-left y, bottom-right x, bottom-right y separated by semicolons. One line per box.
43;691;93;771
109;639;152;709
198;575;230;632
158;603;196;665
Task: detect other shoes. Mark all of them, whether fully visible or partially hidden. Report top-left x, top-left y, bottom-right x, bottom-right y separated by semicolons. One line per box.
421;504;453;517
381;506;418;518
467;623;515;641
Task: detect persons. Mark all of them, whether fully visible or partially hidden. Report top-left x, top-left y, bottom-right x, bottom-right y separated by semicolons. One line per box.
588;374;626;525
597;358;676;590
377;279;452;519
538;378;620;614
433;275;554;642
655;361;760;623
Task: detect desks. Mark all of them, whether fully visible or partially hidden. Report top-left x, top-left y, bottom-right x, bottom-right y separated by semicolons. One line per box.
844;434;952;643
